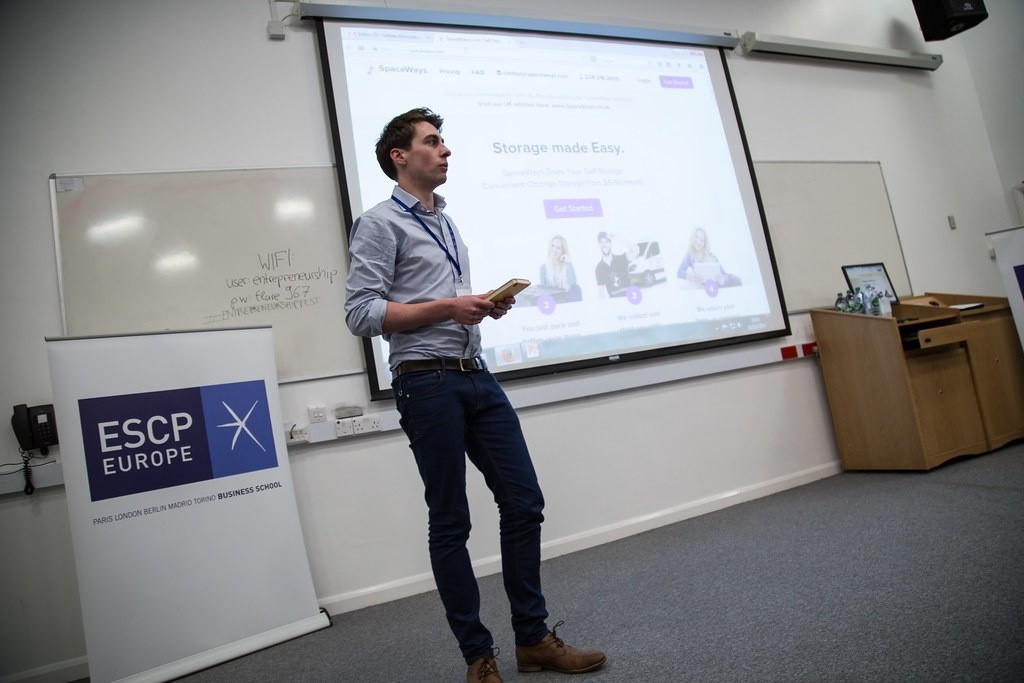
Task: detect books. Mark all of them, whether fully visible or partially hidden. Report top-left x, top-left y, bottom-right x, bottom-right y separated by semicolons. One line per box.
483;279;531;303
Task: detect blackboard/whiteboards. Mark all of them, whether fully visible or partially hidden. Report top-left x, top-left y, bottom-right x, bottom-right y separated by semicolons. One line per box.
48;159;914;388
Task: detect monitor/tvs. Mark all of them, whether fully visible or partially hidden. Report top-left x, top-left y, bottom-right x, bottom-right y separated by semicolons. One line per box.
841;262;900;305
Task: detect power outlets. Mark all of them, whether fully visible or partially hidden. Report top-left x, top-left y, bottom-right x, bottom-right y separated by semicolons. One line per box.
352;416;367;435
803;342;818;356
335;419;351;437
781;345;799;360
369;415;381;432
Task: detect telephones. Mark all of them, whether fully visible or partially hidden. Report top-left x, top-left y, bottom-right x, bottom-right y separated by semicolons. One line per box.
10;402;60;451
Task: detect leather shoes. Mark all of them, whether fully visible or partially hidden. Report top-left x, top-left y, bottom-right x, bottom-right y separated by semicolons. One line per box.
467;647;504;683
516;620;607;672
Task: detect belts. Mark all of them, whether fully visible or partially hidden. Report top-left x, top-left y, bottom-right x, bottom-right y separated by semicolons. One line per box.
392;356;487;379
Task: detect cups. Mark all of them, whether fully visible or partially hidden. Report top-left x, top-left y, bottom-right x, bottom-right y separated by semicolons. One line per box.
878;297;892;318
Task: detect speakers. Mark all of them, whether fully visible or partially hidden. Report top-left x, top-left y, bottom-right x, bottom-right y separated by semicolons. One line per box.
912;0;988;42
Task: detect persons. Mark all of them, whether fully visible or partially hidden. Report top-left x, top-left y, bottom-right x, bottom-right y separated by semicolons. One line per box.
595;231;632;293
539;235;576;292
344;107;607;683
677;226;726;289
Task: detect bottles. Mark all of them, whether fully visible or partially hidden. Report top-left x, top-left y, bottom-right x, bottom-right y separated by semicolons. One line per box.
863;284;881;316
835;292;845;312
845;290;854;313
853;287;866;314
